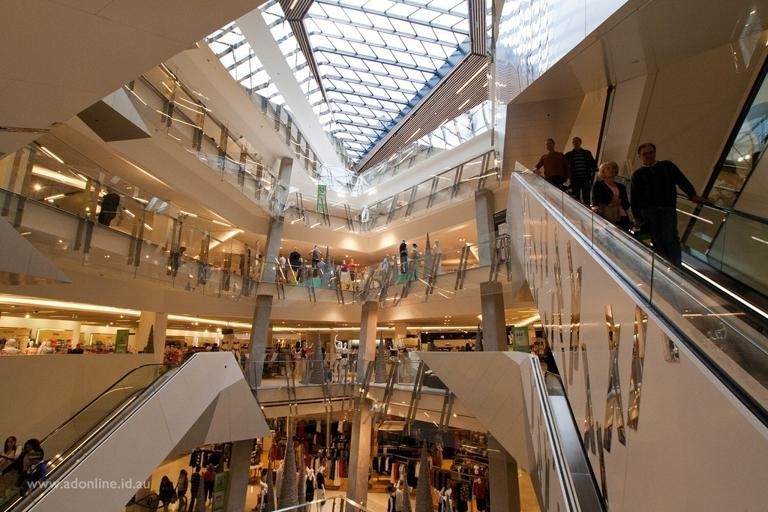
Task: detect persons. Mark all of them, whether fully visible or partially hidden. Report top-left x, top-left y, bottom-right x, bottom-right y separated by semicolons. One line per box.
316;464;327;511
707;161;748;208
531;138;566;191
71;344;84;354
564;137;597;207
66;339;73;354
97;185;120;227
389;344;400;355
628;142;709;270
305;467;315;511
18;447;48;496
0;438;43;486
0;337;55;356
0;435;23;503
589;160;632;234
239;239;442;291
386;486;397;512
289;333;349;384
149;464;217;512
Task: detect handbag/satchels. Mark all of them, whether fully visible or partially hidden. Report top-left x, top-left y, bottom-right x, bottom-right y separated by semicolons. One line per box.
603;206;619;222
